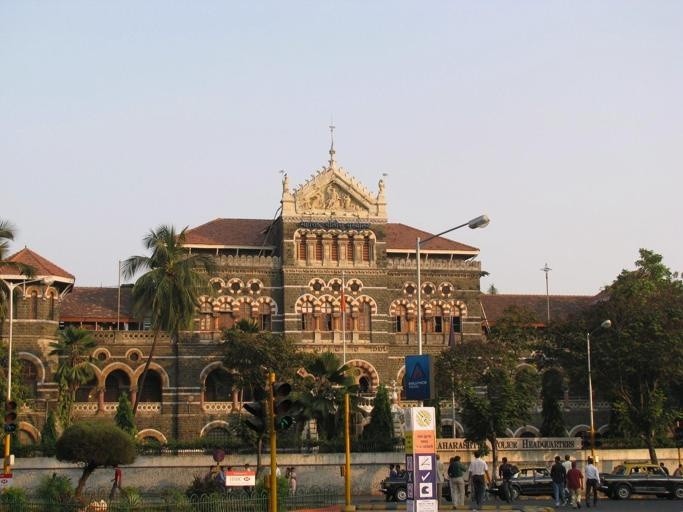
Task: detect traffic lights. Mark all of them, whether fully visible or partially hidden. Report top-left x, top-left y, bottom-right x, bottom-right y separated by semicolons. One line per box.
4;400;18;434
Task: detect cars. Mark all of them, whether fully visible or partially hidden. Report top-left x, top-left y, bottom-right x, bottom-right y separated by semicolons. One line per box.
486;466;571;501
595;459;683;501
379;470;470;502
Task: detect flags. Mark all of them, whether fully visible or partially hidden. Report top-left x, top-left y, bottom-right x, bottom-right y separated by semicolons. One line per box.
448;317;457;347
339;280;347;312
404;354;431;401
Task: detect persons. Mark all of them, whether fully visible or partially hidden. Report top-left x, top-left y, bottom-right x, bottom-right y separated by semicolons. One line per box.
467;450;490;506
435;454;445;509
396;464;403;477
660;462;670;475
282;172;289;193
447;457;454;497
107;464;128;500
551;456;566;509
384;464;396;502
583;458;601;508
325;185;341;209
562;453;573;475
208;463;297;496
673;463;682;478
499;457;515;505
448;456;467;510
566;462;585;509
378;178;386;193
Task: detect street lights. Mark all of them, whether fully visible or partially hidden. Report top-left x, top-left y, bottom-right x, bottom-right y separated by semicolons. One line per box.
587;319;614;466
116;255;137;331
413;213;490;406
2;273;58;497
541;262;552;326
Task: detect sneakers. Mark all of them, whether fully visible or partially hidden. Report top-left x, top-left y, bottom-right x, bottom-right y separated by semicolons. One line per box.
555;500;597;509
